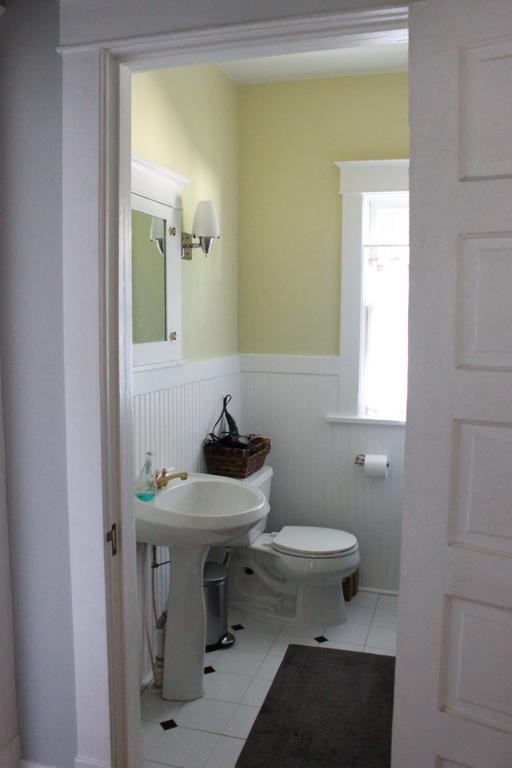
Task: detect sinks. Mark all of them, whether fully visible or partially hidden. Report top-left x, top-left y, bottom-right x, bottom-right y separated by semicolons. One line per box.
133;465;271;546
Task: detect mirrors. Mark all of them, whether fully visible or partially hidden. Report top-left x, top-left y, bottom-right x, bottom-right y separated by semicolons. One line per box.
131;206;167;344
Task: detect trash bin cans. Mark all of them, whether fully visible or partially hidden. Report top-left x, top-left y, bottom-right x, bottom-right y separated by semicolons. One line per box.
203;562;236;651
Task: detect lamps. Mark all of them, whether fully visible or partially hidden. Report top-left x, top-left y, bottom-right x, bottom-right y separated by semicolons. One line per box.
182;199;220;259
149;215;167;255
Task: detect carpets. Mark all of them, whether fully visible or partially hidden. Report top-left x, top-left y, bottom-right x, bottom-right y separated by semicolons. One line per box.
235;644;395;768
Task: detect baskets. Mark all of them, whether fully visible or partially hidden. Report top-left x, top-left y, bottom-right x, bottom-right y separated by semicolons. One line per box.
205;436;270;479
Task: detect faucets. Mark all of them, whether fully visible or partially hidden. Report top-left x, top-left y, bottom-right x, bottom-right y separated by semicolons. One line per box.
147;468;189;491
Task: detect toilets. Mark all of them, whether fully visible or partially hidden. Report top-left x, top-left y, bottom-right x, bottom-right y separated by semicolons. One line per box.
224;464;360;627
